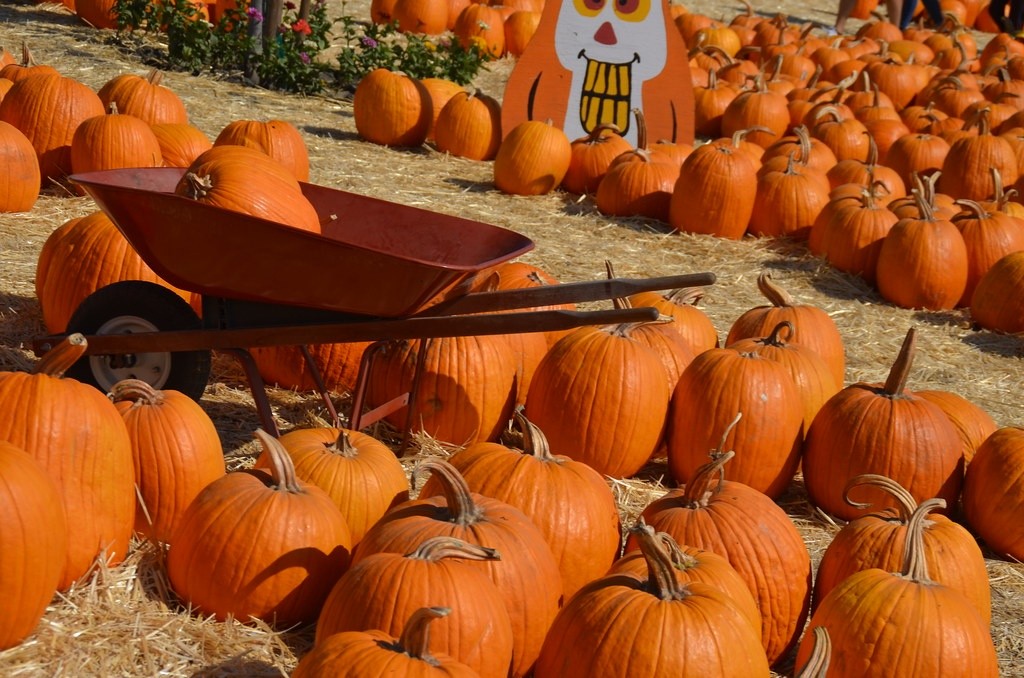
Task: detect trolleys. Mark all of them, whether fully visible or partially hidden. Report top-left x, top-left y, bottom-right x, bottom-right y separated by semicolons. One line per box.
65;165;719;401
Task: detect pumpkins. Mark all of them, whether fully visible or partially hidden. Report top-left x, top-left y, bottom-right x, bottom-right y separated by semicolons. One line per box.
2;0;1023;677
